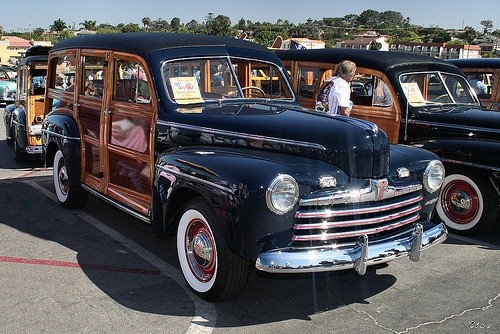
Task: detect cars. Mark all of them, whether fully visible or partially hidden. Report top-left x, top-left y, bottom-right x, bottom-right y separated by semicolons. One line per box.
0;65;17;105
39;31;450;305
270;48;500;238
3;45;52;162
443;58;500;110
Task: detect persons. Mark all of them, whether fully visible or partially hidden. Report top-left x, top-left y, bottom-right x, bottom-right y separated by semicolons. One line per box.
317;60;357;117
469;74;487;93
32;62;240;115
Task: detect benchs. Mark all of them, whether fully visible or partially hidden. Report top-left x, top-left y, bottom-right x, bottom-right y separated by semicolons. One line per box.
90;78;149;105
